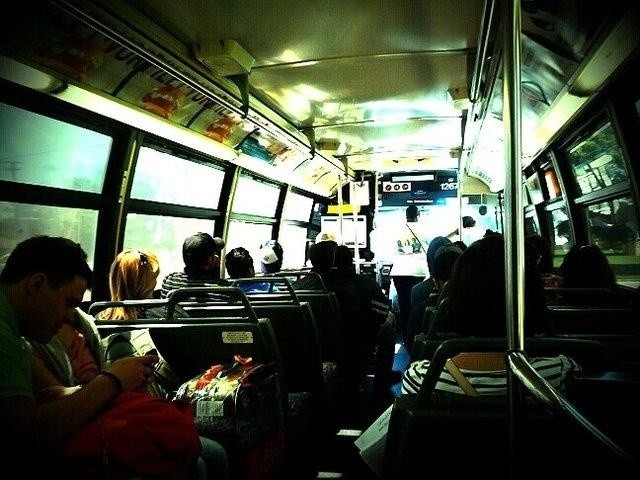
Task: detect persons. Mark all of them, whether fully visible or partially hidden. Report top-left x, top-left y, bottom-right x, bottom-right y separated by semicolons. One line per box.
1;233;616;479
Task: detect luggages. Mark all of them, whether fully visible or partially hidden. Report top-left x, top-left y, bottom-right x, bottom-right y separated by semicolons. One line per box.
171;354;284;480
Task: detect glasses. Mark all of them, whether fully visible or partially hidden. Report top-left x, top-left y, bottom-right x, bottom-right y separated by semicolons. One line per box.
138;251;147;271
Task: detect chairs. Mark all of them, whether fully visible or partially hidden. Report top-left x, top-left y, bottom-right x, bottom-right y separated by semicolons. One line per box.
382;275;640;480
88;268;381;478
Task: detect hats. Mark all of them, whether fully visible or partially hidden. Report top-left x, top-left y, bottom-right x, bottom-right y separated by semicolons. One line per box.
260;240;282;264
183;232;224;257
434;245;462;278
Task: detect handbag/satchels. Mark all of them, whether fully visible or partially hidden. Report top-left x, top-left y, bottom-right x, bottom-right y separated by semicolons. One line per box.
61;391;201;480
